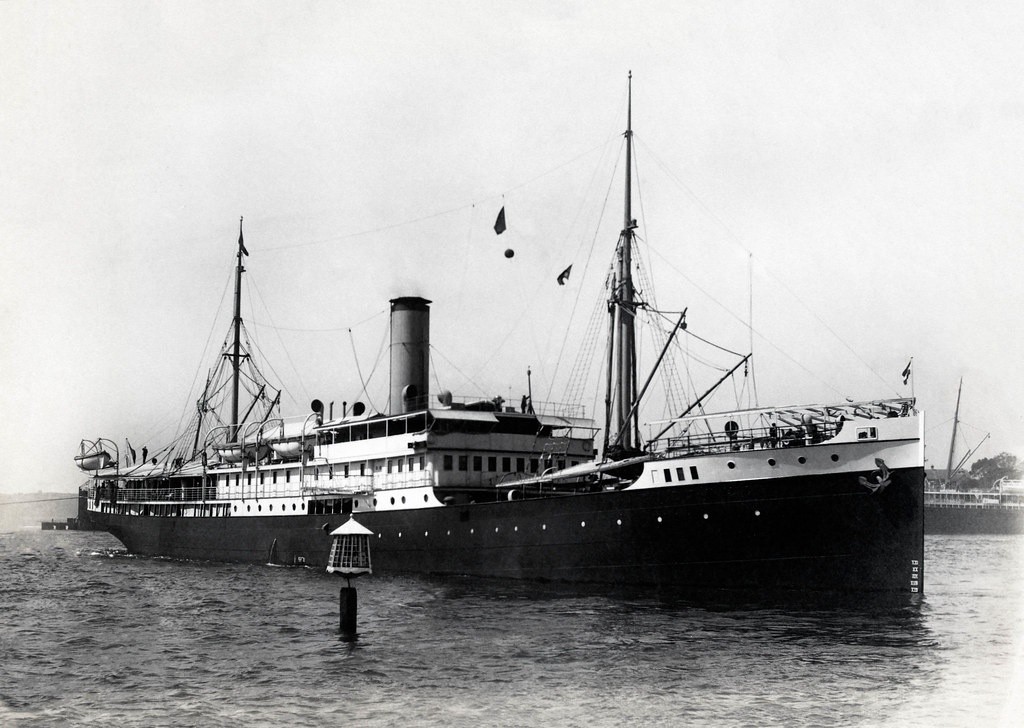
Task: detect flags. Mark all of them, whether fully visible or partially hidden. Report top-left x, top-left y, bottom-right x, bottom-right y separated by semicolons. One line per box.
902;358;912;385
557;263;572;285
494;206;506;235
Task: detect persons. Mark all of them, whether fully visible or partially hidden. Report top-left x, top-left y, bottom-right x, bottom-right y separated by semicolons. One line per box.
521;395;530;413
142;446;148;464
101;480;128;498
770;423;777;448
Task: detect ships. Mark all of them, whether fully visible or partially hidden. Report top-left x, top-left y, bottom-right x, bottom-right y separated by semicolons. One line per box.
70;71;925;615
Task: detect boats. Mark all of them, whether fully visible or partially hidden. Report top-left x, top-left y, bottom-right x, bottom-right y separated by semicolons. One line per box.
495;451;651;497
74;449;109;470
210;440;269;462
270;442;304;459
